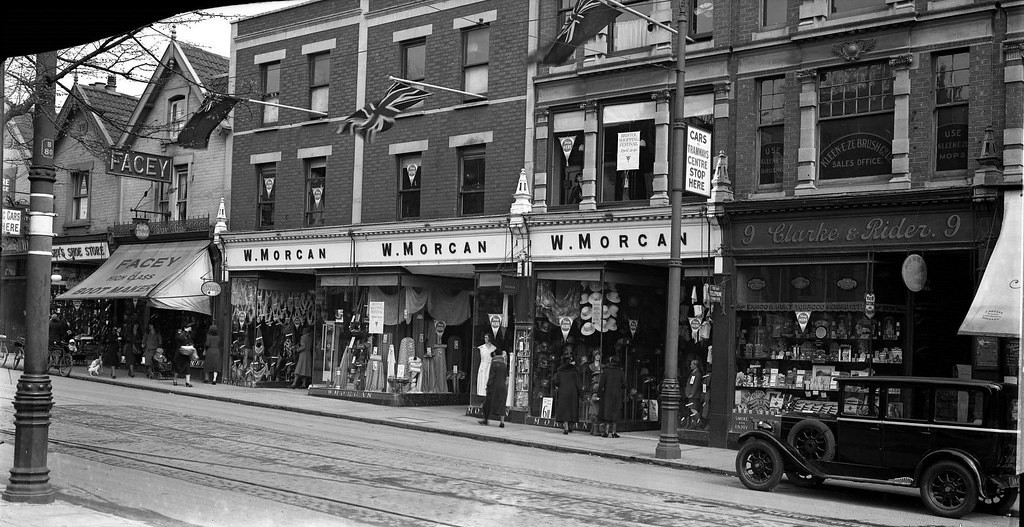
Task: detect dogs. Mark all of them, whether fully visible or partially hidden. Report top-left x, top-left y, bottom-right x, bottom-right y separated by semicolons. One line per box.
86;355;104;376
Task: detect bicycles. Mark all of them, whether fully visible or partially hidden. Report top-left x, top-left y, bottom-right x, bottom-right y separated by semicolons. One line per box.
47;341;74;377
0;334;8;367
12;336;24;369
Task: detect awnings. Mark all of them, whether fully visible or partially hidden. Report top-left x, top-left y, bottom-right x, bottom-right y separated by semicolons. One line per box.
957;189;1022;336
56;241;214;315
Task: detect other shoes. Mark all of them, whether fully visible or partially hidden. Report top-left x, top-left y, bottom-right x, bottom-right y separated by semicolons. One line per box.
128;370;135;377
202;379;209;383
499;423;504;427
212;381;216;384
286;385;296;389
299;386;306;389
172;381;177;385
479;420;487;424
111;372;116;379
186;383;192;387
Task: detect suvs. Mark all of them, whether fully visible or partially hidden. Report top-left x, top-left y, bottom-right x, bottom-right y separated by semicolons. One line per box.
733;376;1018;518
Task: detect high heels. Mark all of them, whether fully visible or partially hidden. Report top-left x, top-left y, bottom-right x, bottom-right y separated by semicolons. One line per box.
612;433;620;438
602;431;609;437
568;426;573;432
563;429;568;435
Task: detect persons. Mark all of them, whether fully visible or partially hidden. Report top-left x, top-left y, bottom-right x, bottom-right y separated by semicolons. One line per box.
290;328;312;389
587;350;604;388
569;173;582;204
686;360;701;413
557;355;580;434
49;312;74;361
579;356;592;386
476;333;497;396
142;323;172;379
172;323;193;387
478;348;508;428
599;356;625;437
122;337;141;377
104;324;121;377
202;325;222;385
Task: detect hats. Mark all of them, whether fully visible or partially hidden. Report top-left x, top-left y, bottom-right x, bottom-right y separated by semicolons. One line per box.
580;281;620;335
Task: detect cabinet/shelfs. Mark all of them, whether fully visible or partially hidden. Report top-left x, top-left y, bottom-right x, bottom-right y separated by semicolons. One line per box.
733;309;905;424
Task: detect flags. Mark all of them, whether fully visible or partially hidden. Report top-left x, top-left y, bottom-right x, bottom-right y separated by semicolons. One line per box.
178;93;249;149
544;0;622;66
337;78;432;142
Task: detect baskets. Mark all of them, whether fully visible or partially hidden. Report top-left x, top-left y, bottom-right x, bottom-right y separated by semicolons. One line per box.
179;344;194;355
